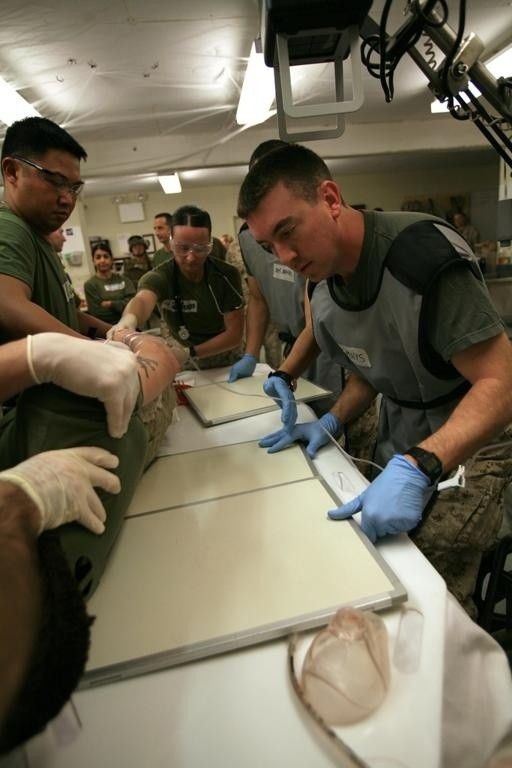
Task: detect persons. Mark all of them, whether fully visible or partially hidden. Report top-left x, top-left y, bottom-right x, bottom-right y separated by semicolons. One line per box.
453;212;479;250
0;117;114;345
0;331;140;712
236;144;512;621
47;204;282;370
228;139;379;481
0;326;181;751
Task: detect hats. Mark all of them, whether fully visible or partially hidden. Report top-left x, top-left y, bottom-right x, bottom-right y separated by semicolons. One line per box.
128;235;149;252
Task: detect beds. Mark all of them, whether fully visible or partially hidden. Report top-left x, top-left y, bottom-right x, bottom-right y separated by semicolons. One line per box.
2;358;512;764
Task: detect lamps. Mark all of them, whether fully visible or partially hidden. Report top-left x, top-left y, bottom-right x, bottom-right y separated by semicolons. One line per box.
427;36;512;115
235;38;278;124
157;170;182;194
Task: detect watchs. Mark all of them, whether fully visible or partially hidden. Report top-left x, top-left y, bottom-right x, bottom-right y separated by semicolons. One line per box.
268;370;297;392
404;446;443;483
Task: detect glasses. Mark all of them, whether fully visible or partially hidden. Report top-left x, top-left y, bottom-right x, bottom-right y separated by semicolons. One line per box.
170;236;214;259
12;154;84;197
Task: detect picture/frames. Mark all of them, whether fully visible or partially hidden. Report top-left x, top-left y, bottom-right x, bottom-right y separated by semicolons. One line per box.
142;233;156;254
